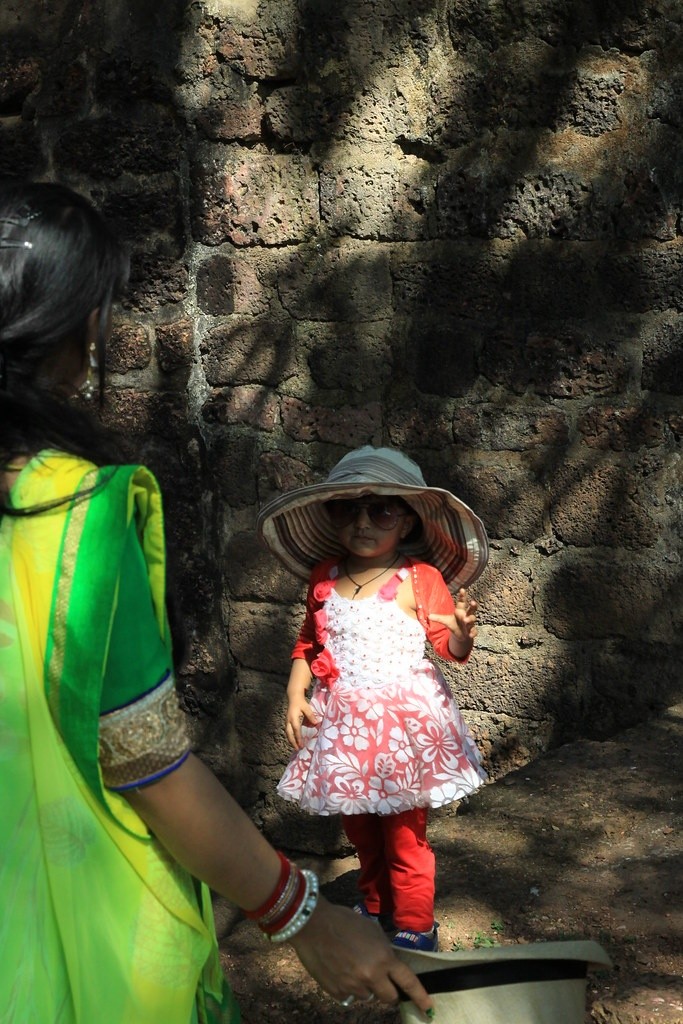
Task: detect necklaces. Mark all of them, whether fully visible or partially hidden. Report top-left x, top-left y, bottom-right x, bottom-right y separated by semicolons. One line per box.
344;550;401;600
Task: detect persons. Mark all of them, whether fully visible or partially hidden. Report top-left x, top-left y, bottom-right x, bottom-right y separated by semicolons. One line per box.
0;180;437;1024
258;443;491;956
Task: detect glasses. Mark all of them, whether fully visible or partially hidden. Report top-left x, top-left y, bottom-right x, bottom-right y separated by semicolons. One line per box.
328;496;408;530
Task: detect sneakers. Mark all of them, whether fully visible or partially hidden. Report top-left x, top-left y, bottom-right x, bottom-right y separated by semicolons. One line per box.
351;902;391;930
391;922;441;952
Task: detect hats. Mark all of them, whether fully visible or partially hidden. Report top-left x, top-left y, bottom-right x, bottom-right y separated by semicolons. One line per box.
388;939;615;1024
255;445;490;595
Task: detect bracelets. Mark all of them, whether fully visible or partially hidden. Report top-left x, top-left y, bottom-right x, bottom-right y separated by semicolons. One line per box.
241;849;320;944
